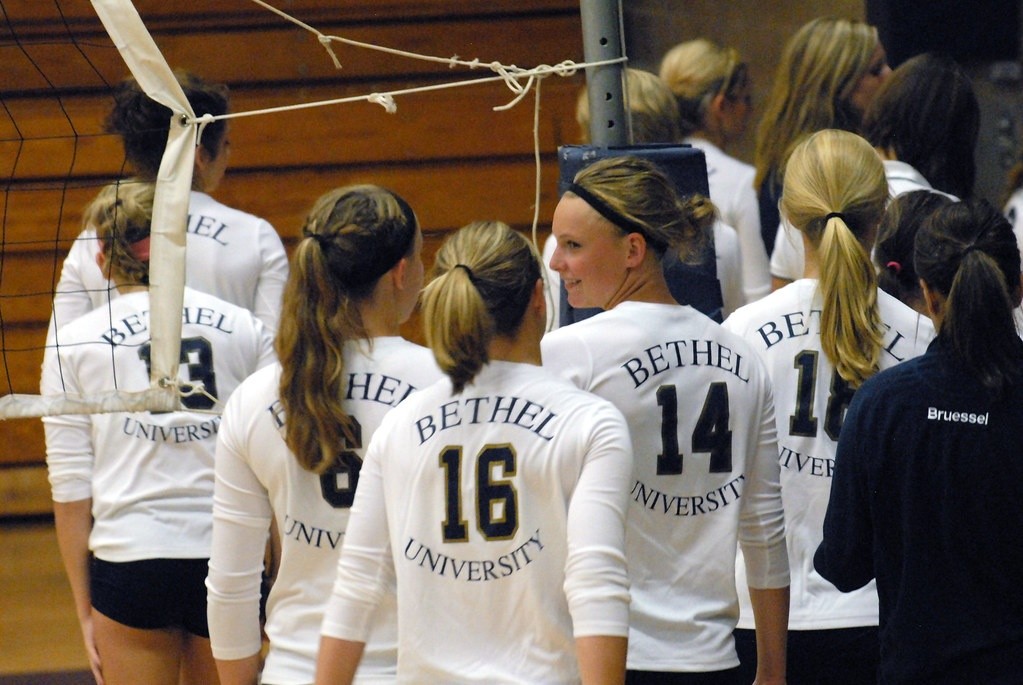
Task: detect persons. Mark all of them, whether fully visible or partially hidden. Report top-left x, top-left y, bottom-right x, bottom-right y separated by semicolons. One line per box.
752;17;892;264
48;70;290;339
811;199;1023;685
207;184;445;685
769;54;982;294
721;129;937;685
870;189;951;315
659;39;771;303
41;177;277;685
316;220;633;685
539;67;741;334
540;156;791;685
